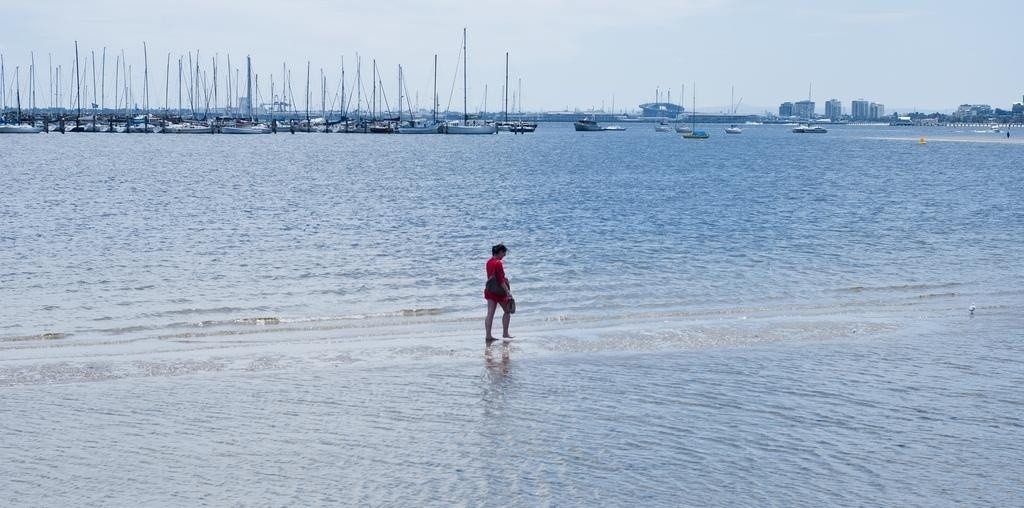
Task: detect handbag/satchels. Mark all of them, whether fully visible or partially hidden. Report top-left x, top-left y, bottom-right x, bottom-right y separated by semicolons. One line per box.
484;260;511;297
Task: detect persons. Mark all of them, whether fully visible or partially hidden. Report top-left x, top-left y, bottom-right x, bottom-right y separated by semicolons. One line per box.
484;243;515;341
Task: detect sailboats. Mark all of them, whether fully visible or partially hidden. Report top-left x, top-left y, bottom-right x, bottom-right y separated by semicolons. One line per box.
572;83;829;140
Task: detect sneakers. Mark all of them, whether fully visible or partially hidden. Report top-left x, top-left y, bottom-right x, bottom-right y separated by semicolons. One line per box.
504;298;516;314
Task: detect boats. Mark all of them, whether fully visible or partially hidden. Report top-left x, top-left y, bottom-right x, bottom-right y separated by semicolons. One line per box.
990;122;1001;132
